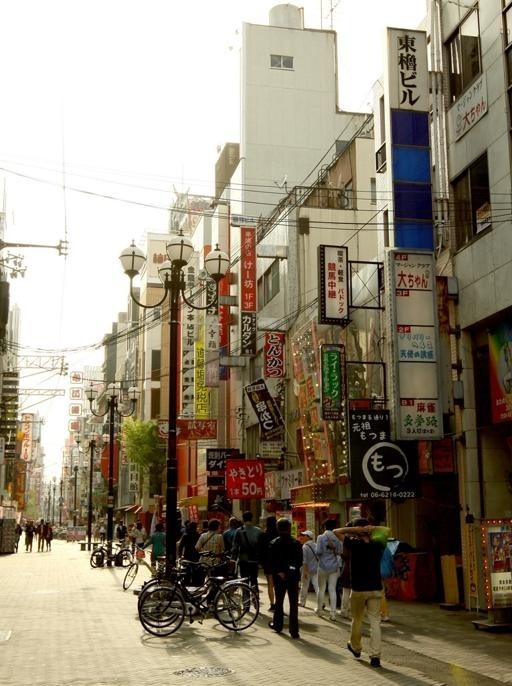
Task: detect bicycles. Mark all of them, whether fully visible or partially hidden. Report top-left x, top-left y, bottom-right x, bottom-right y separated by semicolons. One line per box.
85;534;133;571
135;547;259;636
123;543;173;591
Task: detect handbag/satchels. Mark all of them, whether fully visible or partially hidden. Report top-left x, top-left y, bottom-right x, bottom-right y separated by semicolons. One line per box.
246;542;260;562
380;547;395;578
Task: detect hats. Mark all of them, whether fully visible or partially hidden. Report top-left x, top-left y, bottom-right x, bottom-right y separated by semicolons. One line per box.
300;530;314;538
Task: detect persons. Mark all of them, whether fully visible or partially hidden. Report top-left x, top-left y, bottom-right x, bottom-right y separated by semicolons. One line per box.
261;515;286;611
200;520;209;534
314;523;343;621
16;514;54;552
222;518;242;579
196;521;224;574
299;528;326;612
267;520;305;640
96;518;167;575
336;517;396;668
178;521;201;563
233;512;267;612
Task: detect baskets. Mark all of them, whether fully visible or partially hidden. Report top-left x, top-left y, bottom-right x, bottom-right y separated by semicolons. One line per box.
216;561;235;576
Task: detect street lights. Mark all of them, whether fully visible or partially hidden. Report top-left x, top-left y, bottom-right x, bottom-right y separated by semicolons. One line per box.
78;371;143;566
40;431;111;543
106;236;232;592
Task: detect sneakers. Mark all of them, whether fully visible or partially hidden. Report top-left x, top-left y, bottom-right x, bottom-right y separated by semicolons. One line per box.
268;621;283;632
292;632;299;639
370;657;380;667
268;605;275;612
347;640;361;658
298;602;350;621
382;616;391;622
